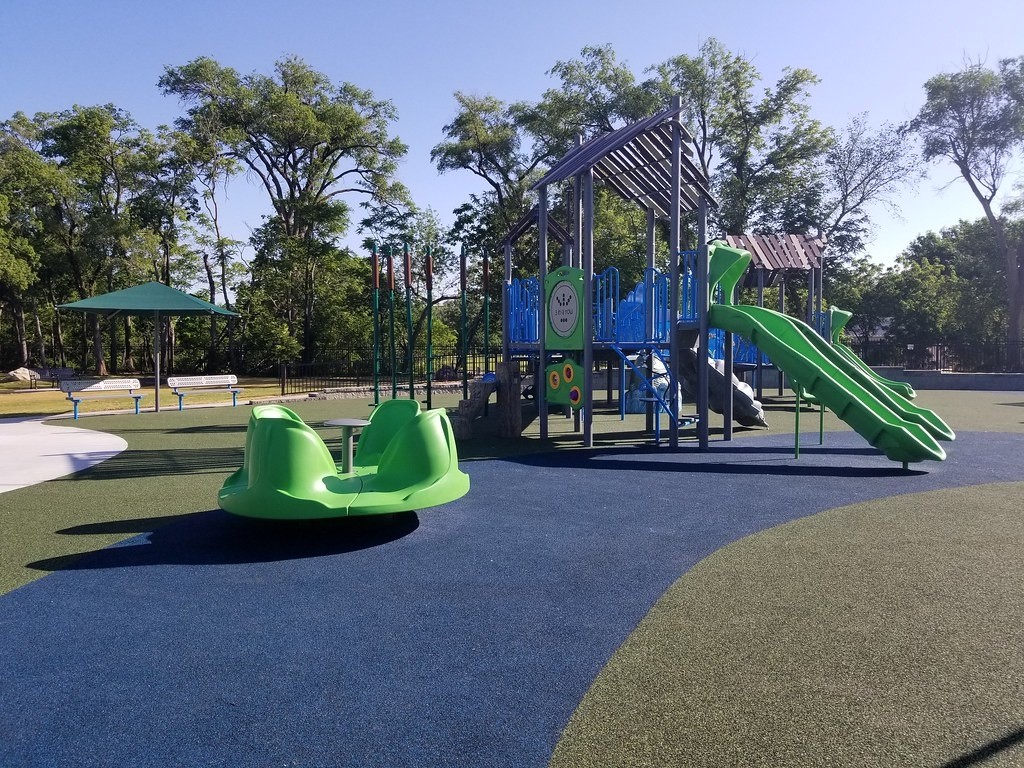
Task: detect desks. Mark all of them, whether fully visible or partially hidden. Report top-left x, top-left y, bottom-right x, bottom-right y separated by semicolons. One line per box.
323;419;371;472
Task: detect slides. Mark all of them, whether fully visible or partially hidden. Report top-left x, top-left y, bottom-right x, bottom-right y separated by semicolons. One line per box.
712;301;958;463
833;343;917;401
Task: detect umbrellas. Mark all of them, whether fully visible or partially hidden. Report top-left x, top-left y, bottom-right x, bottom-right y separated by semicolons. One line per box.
56;281;239;412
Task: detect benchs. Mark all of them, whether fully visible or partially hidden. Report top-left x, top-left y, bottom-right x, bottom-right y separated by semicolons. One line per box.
167;374;240;411
60;379;143;419
49;368;81;387
29;367;55;389
